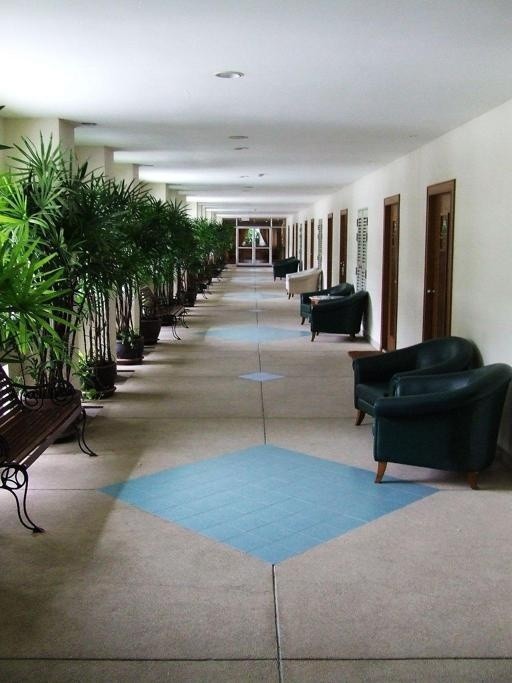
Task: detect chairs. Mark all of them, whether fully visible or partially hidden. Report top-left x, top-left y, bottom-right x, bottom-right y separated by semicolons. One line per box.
352;335;511;491
271;256;367;341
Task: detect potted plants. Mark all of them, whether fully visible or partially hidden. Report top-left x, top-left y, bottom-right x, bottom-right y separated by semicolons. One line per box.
162;197;233;325
79;169;162;398
1;129;95;443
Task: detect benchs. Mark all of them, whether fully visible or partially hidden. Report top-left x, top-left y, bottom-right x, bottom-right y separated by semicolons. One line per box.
194;260;222;301
139;287;189;340
1;366;97;535
